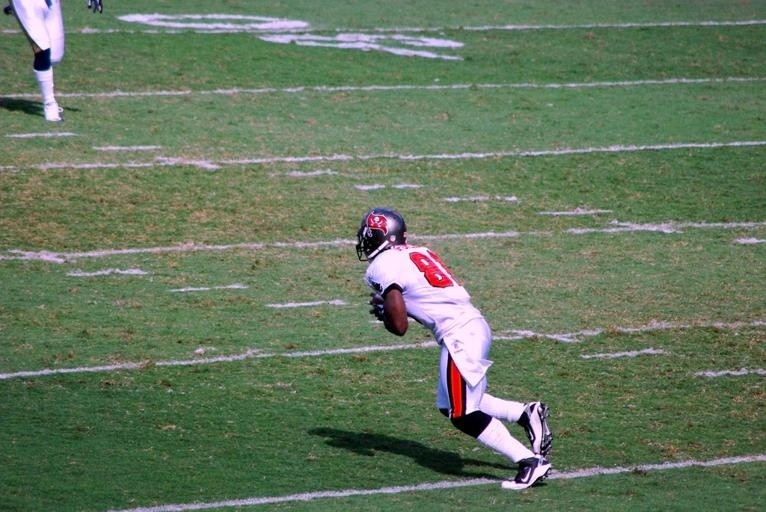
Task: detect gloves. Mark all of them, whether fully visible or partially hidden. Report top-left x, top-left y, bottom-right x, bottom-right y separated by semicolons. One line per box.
87;0;103;15
369;304;384;322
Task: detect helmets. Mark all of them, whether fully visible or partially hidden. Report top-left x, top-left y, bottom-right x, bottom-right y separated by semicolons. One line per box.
361;207;407;251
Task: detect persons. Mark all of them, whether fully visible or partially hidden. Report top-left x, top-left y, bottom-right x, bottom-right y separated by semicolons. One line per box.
1;0;104;125
354;204;554;491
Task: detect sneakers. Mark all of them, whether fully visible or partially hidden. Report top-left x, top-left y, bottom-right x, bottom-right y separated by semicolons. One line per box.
3;5;14;15
42;101;64;123
516;401;554;459
501;454;552;490
